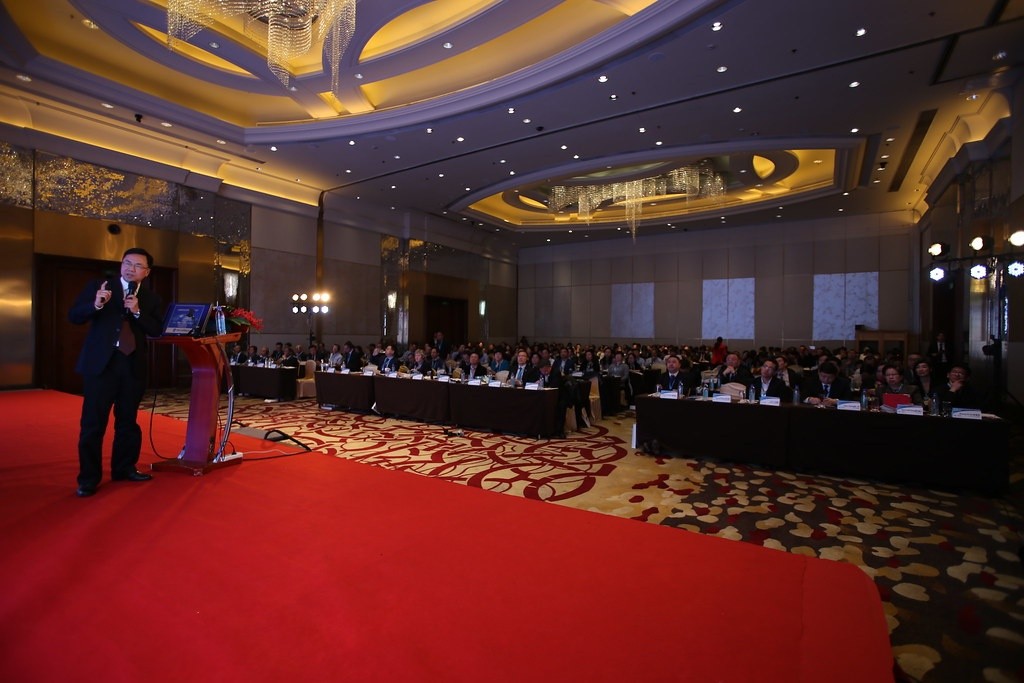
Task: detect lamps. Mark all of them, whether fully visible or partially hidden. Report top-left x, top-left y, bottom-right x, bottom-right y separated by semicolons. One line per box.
291;293;331;315
167;0;356;95
548;160;728;243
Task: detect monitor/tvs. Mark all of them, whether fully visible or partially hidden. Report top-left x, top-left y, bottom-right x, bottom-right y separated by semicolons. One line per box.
161;302;214;337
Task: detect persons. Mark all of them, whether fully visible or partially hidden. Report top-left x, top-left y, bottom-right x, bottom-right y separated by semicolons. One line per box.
76;249;161;496
330;332;978;430
230;342;328;396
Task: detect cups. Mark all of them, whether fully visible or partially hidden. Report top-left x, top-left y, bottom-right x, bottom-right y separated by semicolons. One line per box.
385;368;390;377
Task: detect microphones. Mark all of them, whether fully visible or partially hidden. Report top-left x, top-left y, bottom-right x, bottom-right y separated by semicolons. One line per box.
126;281;138;313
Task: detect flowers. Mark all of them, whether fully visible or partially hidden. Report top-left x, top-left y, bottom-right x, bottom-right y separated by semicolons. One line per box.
212;306;264;334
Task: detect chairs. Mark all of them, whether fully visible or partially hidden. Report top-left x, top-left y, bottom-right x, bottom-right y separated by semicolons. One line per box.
296;360;316;399
587;375;602;424
563;406;578;433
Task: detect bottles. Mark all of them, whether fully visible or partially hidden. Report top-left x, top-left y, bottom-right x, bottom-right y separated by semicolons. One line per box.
510;376;515;388
703;389;708;401
679;386;683;395
709;374;714;391
265;358;272;368
461;370;465;383
931;393;939;416
749;384;755;403
413;366;417;376
793;385;800;405
437;368;440;379
342;362;346;371
861;389;868;410
216;307;226;335
540;378;544;388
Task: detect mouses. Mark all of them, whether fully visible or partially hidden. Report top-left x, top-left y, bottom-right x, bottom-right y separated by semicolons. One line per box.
193;332;206;339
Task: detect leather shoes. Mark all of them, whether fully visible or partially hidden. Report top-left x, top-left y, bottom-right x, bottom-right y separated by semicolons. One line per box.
77;484;96;496
112;467;151;481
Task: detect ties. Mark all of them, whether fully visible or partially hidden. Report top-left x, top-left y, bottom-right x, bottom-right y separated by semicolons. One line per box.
824;384;828;397
117;290;136;357
670;375;675;387
384;358;389;368
938;344;943;360
516;367;523;380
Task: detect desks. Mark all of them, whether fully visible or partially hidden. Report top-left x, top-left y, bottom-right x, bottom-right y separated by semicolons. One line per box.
229;362;1008;493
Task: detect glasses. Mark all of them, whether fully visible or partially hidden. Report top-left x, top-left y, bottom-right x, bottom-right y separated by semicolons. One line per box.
121;260;150;271
518;355;526;357
763;364;773;372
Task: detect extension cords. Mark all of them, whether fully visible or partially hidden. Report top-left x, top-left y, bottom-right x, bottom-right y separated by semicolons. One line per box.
219;452;243;462
